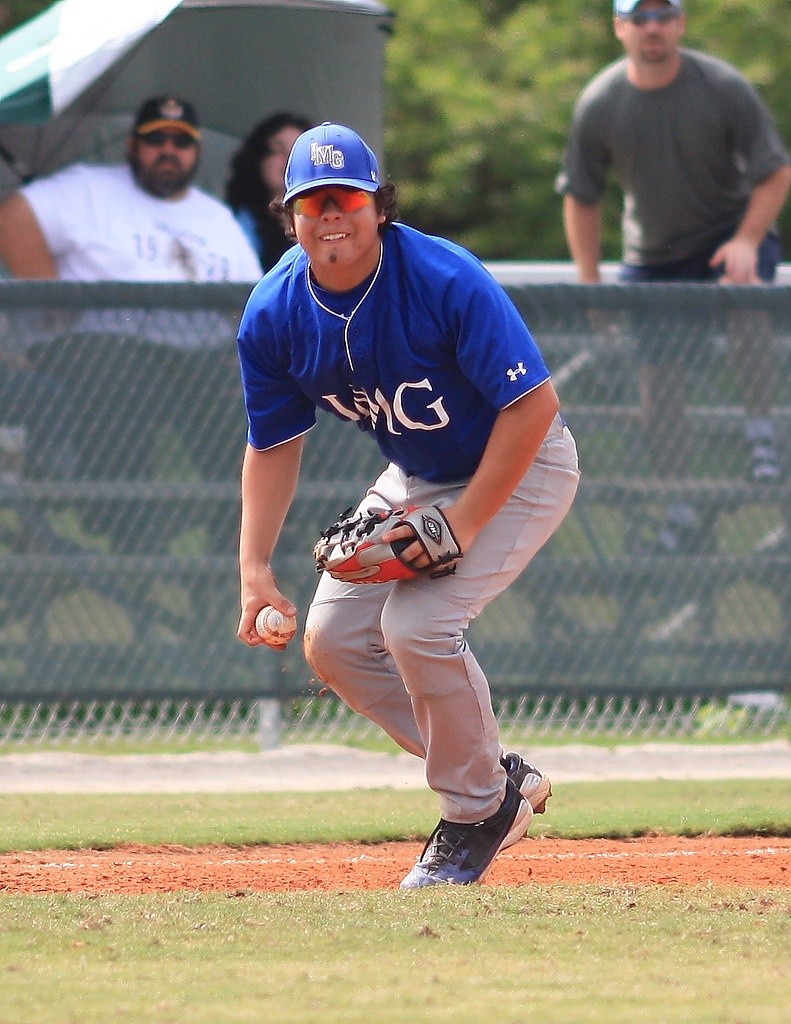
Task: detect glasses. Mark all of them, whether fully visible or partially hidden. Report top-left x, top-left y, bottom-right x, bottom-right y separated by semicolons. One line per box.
292;190;371;218
619;10;677;26
144;131;195;147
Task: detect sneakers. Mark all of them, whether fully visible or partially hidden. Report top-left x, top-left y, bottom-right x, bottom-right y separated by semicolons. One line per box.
399;776;534;891
498;754;551;808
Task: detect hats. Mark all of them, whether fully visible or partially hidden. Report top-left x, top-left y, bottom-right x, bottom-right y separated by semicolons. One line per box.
136;94;200;140
615;0;678;13
280;122;380;204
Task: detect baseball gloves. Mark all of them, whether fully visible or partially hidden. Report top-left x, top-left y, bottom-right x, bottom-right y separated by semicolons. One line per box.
313;501;426;585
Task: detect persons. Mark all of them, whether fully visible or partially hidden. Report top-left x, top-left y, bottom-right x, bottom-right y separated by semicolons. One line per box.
237;120;581;889
223;113;320;270
0;94;297;555
555;0;791;550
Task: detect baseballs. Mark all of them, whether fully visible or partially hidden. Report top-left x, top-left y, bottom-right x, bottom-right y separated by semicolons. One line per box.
253;603;294;647
